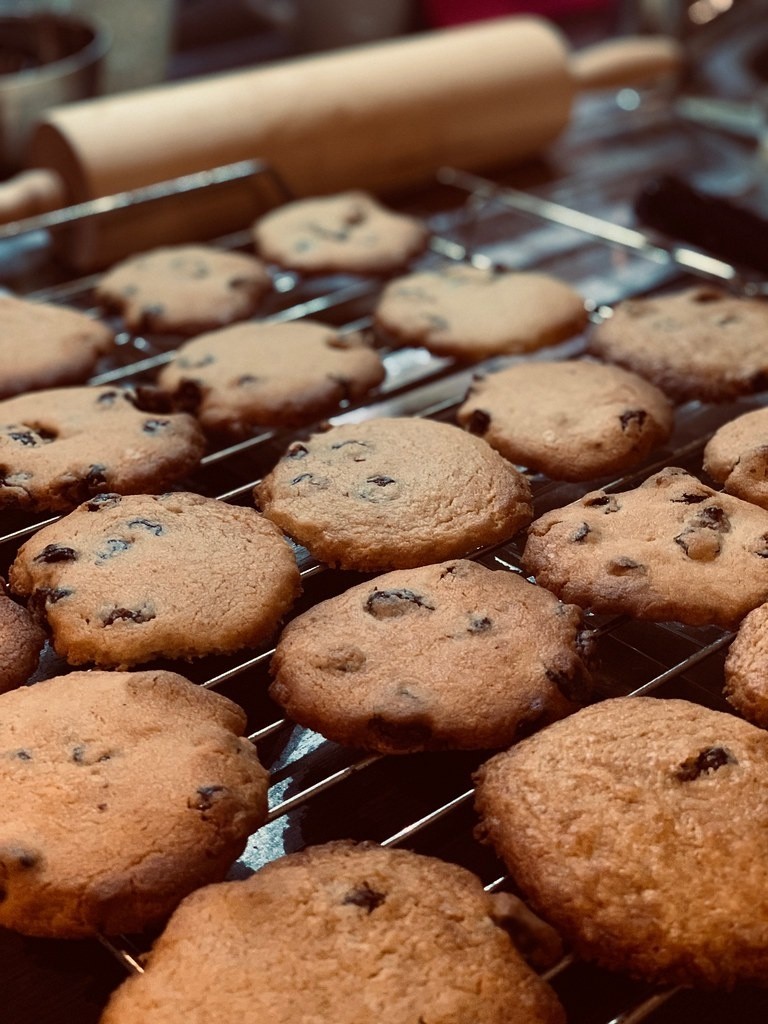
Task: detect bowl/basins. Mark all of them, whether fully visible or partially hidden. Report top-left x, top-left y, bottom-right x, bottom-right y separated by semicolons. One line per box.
0;13;111;169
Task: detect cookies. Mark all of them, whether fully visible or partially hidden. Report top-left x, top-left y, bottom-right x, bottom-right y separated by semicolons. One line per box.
0;190;768;1024
